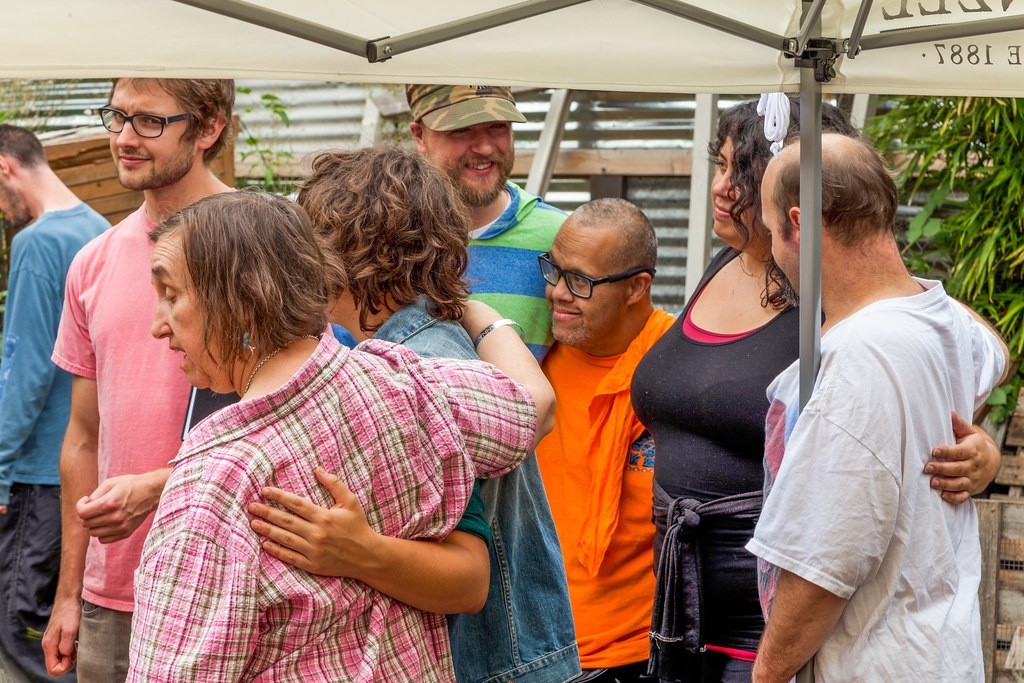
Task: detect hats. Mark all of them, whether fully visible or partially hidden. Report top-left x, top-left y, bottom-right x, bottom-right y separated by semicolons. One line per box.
406;84;527;131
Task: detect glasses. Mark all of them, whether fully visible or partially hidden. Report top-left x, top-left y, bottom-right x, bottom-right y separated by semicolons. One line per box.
538;252;655;299
98;104;193;138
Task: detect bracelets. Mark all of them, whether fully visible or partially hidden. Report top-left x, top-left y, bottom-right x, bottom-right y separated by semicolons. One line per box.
474;320;527;355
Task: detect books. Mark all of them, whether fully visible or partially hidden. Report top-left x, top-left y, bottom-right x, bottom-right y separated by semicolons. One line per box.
181;385;241;436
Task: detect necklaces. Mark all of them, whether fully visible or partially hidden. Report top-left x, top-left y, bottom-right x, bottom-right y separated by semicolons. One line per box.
244;335;320;399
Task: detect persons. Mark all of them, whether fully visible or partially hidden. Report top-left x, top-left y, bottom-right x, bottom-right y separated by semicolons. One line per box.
534;197;682;683
0;124;116;683
249;148;586;683
743;131;1010;682
39;78;238;683
406;82;576;375
630;92;1004;683
124;191;559;683
